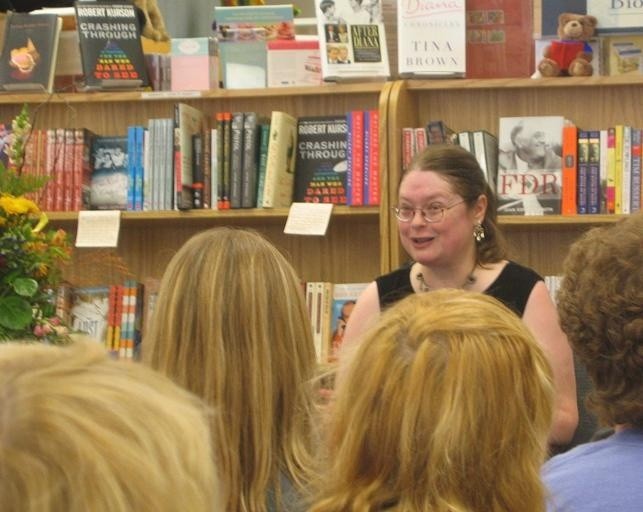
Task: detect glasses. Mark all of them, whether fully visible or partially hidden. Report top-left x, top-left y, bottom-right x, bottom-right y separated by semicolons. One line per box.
390;193;479;224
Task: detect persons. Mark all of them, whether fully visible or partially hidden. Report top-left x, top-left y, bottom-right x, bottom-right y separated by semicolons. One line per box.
316;144;579;447
138;226;322;510
289;287;557;511
0;333;228;511
537;213;643;512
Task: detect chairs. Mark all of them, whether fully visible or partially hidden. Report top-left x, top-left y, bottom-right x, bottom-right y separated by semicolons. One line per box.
567;337;616;445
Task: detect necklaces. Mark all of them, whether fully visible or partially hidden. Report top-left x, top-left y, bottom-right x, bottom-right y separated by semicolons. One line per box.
415;266;479;292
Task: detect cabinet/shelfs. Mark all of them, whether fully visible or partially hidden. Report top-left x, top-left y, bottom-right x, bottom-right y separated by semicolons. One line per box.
0;83;387;386
389;76;640;346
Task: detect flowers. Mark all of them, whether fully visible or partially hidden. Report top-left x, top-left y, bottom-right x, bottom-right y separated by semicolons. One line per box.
0;73;140;346
